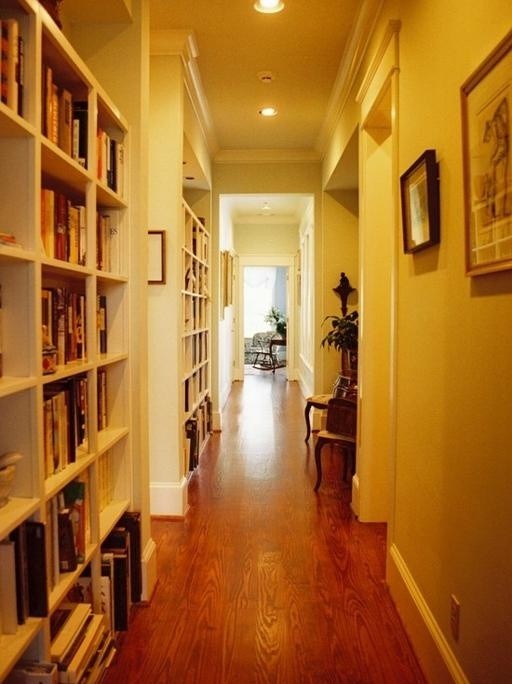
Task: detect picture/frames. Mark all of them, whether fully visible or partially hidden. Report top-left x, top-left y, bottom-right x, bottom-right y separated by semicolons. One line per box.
399;27;511;276
148;229;167;284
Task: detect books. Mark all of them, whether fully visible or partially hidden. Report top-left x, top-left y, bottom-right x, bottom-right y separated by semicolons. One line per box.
41;188;112;272
99;451;113;511
2;452;23;508
1;233;22;248
1;16;126;198
0;478;88;634
185;218;213;477
43;371;110;480
41;290;107;373
0;511;142;683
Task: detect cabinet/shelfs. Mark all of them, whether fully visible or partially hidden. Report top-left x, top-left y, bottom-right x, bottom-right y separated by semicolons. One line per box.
182;129;213;485
0;0;139;684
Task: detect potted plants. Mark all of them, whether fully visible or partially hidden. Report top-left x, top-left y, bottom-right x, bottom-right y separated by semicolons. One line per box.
319;310;358;370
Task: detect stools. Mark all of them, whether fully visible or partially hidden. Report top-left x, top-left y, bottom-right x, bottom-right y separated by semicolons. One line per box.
304;393;333;442
313;430;356;490
